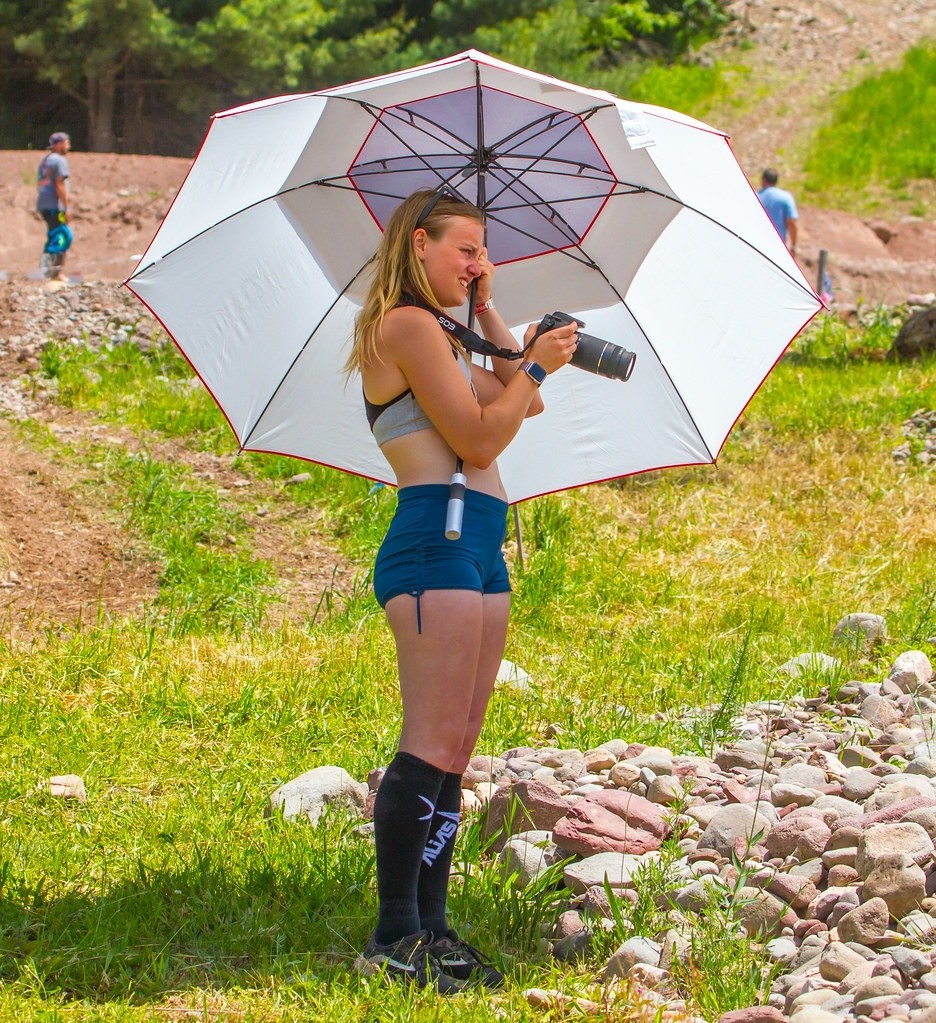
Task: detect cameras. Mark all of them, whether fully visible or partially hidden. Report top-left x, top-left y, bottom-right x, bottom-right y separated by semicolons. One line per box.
537;312;636;383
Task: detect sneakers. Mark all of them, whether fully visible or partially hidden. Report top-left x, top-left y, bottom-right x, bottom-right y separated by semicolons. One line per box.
429;929;506;990
356;930;478;997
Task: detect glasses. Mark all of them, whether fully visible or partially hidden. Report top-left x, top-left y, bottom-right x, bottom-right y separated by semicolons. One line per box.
415;186;466;229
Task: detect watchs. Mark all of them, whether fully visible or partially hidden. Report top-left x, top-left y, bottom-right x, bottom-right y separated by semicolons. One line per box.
518;361;548;388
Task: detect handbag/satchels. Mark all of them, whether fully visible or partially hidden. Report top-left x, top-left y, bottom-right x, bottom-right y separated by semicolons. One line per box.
43;223;74;256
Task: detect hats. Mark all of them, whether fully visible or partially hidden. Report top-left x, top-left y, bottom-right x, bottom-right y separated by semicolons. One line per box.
47;132;68;150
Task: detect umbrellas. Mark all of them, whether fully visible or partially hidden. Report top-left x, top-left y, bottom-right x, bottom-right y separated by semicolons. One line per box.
117;46;831;540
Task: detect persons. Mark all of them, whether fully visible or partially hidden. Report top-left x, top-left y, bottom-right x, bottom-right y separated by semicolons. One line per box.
342;190;578;998
36;132;73;281
757;168;800;257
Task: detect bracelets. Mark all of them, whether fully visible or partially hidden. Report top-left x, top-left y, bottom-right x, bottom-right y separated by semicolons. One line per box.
475;297;494;316
